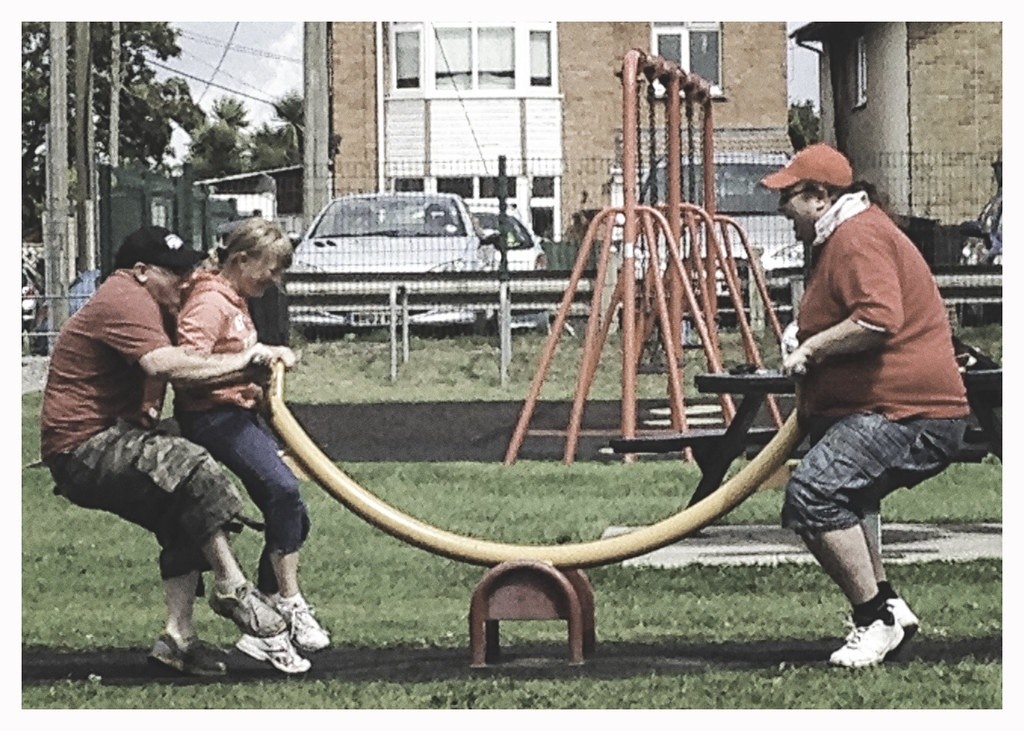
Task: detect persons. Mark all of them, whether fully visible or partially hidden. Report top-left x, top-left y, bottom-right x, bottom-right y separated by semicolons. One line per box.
759;145;971;668
172;221;331;653
39;225;297;673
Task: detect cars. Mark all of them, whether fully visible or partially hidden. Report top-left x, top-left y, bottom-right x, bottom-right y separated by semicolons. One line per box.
620;150;805;299
469;204;548;328
283;191;485;340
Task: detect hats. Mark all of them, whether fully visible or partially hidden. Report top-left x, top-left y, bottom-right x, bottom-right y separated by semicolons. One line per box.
117;225;210;267
760;142;854;189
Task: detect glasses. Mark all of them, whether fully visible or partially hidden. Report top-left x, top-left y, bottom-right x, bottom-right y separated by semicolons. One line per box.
778;182;815;205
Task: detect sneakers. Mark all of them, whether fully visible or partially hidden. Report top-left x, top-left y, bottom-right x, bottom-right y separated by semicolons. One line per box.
150;631;227;676
271;592;330;652
208;579;288;638
885;595;921;659
235;630;312;673
827;606;907;668
